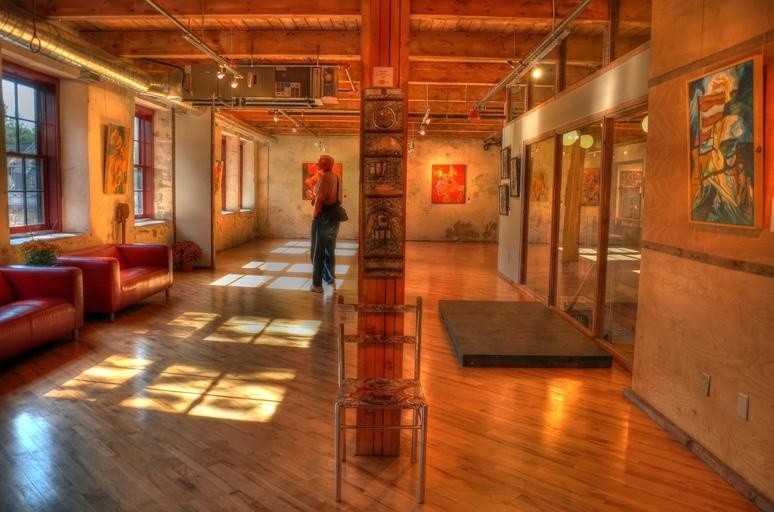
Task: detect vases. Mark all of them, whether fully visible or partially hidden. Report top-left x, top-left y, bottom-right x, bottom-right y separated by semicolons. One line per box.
180;259;194;271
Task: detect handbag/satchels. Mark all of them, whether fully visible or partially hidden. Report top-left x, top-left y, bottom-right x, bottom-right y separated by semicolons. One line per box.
327;204;349;223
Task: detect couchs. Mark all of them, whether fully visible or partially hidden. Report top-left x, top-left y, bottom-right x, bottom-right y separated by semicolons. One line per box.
0;258;87;364
57;238;175;322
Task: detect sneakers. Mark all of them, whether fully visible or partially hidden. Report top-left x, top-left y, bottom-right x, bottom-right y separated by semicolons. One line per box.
309;284;323;293
327;281;336;290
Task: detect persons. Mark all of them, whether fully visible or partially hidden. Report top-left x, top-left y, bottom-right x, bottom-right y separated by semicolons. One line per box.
309;154;342;293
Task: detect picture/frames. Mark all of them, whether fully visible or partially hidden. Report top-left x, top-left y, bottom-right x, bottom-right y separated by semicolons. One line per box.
508;157;520;197
497;184;509;217
683;53;764;233
498;147;509;180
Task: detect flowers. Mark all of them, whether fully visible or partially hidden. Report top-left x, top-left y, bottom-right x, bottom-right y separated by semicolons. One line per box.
169;238;203;263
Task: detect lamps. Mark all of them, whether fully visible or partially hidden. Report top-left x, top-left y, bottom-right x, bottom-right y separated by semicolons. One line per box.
273;109;329;151
212;60;243;90
407;106;431;154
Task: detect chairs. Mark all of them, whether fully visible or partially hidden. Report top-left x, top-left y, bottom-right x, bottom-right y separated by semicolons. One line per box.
327;294;429;504
